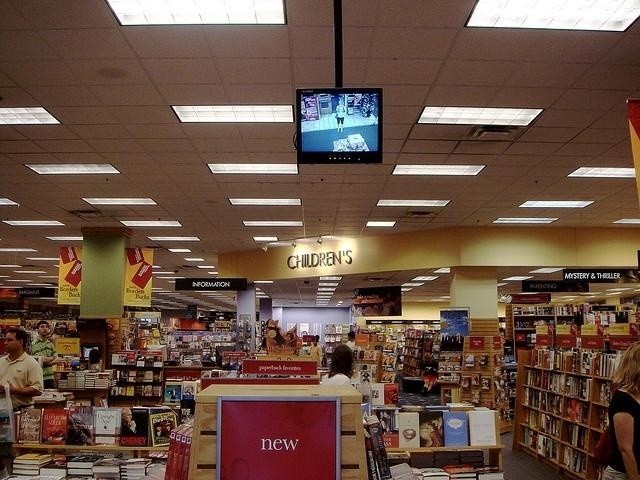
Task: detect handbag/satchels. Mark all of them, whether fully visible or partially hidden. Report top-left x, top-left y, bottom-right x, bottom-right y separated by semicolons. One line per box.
0;384;16;443
595;428;612;462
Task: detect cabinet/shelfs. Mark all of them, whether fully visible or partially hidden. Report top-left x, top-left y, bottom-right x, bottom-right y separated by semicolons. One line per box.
0;283;639;480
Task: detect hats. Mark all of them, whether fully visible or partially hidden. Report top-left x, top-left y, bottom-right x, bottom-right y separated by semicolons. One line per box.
90;349;100;364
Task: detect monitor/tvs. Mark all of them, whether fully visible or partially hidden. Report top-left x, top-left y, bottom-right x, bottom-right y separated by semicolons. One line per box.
295;86;384;165
80;344;99;359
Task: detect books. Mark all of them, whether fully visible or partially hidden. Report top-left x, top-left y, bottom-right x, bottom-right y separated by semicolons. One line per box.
11;318;240;480
524;348;598;472
600;352;624;426
514;307;640;351
360;382;506;480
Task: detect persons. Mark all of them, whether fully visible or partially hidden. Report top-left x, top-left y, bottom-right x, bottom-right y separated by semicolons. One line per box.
321;345;355;384
336;100;346;133
0;329;43;478
315;335;323;347
285;333;297;348
601;341;640;480
419;353;439;391
346;331;355;348
310;337;322;361
